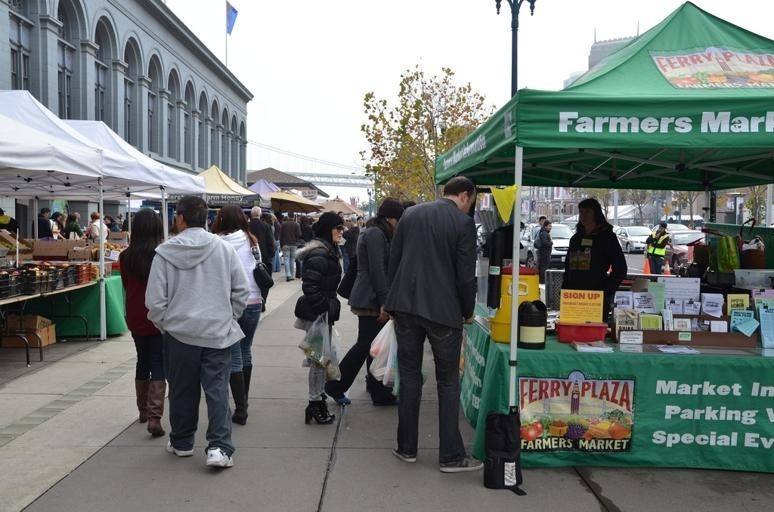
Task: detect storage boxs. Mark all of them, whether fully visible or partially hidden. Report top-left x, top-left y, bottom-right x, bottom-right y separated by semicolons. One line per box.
0;314;55;348
700;269;774;294
32;230;125;277
552;319;608;346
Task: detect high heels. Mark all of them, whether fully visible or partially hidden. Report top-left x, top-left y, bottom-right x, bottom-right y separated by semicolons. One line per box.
303;397;334;425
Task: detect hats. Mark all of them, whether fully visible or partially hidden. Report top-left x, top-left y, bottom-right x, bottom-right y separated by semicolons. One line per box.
312;212;343;235
379;198;403;218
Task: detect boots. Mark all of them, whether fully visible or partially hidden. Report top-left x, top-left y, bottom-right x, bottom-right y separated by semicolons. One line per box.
133;363;253;435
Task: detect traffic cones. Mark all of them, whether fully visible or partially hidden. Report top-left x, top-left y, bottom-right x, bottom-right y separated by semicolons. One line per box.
642;258;652;274
662;259;672;275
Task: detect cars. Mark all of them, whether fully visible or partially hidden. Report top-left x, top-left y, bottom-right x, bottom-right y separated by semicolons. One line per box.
657;230;705;275
519;223;574;270
473;222;690;254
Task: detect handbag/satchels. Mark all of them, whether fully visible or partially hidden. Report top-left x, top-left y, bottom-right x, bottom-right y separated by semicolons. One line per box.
254;264;274;289
737;238;766;268
708;235;740;273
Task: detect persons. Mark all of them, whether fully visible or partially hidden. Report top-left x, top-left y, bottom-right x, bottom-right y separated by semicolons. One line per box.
291;211;345;425
380;176;486;473
207;203;264;424
645;221;670;280
323;195;407;405
0;195;556;281
142;194;251;467
117;208;171;434
560;197;628;324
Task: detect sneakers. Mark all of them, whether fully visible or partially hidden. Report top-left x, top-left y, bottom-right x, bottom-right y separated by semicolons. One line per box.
392;448;483;472
335;390;351;406
166;441;235;468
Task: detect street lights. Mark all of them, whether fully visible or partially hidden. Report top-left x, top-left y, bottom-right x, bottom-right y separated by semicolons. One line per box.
366;185;375;218
426;120;445;201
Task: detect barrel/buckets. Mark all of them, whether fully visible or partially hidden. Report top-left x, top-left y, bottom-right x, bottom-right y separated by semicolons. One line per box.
489;264;540;345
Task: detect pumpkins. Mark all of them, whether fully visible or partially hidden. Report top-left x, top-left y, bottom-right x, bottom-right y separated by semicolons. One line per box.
608;416;632;440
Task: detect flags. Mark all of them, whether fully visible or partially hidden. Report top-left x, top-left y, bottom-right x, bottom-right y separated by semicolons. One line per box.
225;1;237;34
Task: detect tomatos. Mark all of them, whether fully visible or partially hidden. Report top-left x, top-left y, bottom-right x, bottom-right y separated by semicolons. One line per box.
520;422;544;441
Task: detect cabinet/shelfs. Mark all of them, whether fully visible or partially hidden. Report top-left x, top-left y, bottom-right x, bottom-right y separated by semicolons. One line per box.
0;278;105;364
454;295;773;483
32;271;127;340
